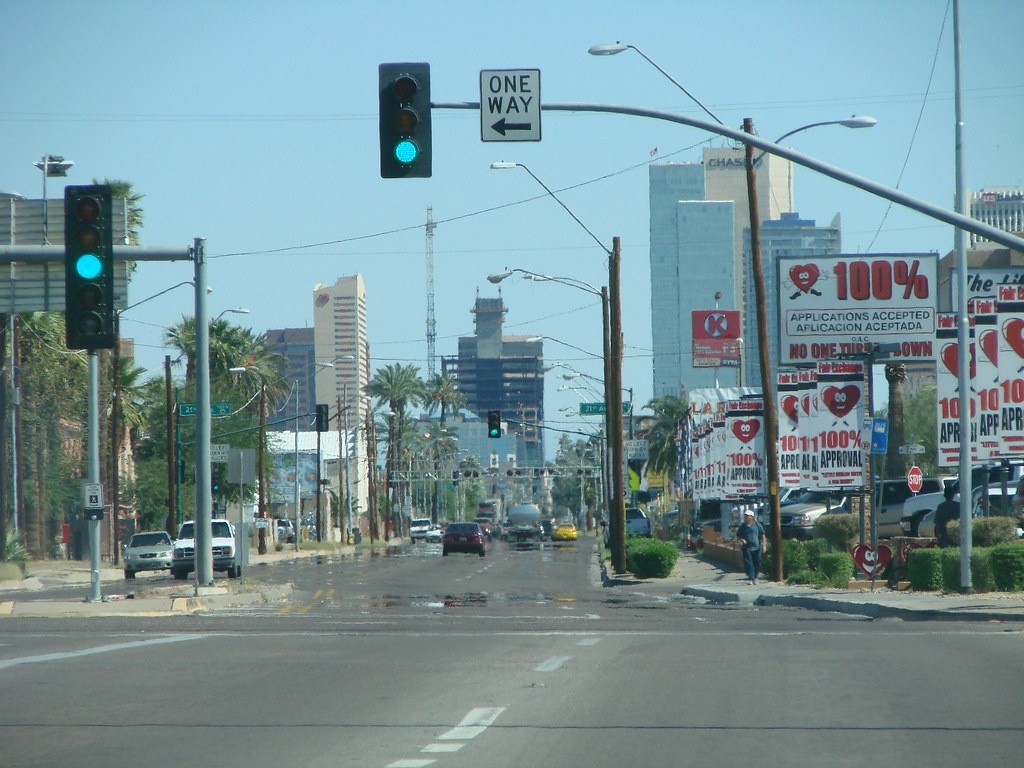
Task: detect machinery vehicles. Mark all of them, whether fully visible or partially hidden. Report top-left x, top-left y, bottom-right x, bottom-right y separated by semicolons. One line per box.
507;502;544;551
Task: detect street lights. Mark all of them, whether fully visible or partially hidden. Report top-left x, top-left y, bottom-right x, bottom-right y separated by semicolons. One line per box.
33;152;75;309
482;160;634;576
85;280;212;606
257;353;397;557
161;309;251;536
584;38;877;584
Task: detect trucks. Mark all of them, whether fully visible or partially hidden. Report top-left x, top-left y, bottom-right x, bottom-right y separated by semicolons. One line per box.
474;500;502;539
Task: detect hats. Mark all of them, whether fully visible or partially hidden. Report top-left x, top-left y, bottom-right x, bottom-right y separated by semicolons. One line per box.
744;510;754;516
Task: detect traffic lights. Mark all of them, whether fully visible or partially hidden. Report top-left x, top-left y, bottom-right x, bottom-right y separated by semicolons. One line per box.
64;185;114;350
487;410;502;438
378;62;433;178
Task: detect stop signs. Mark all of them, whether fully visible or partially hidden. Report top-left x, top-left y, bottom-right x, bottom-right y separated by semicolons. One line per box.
907;466;924;493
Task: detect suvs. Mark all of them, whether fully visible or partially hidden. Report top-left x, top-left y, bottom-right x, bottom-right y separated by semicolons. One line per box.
409;518;433;542
172;518;239;580
277;519;296;543
661;460;1023;548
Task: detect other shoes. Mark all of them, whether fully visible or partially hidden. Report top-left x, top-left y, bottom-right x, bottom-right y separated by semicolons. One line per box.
747;580;753;585
753;578;758;586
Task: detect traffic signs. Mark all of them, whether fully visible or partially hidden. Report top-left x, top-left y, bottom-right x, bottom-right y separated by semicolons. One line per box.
480;69;542;142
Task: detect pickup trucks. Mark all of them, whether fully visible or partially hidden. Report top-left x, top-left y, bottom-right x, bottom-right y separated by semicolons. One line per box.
599;507;651;546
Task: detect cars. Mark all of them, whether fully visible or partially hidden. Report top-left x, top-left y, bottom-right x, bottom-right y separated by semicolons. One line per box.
425;524;443;542
440;517;494;557
123;530;174;579
543;517;578;541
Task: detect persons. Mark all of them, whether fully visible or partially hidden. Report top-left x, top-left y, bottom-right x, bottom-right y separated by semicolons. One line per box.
934;487;960;549
736;511;766;585
